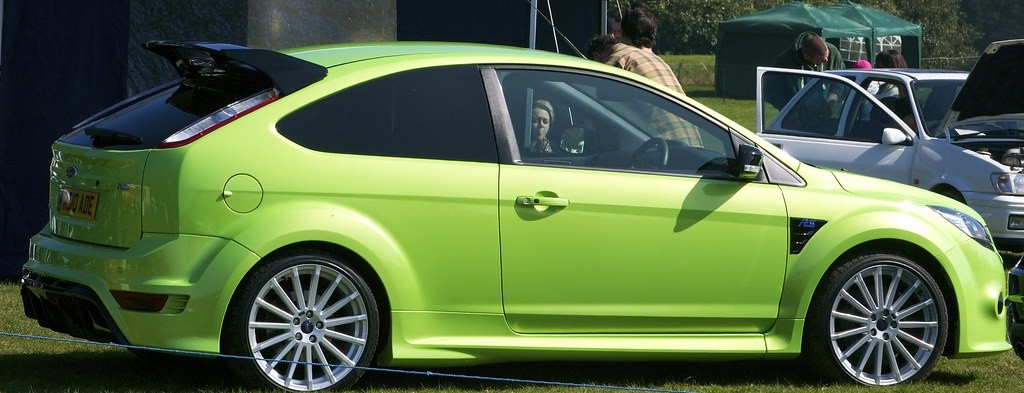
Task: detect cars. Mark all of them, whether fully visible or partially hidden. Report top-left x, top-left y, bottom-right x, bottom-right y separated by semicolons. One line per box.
754;39;1024;255
22;40;1012;393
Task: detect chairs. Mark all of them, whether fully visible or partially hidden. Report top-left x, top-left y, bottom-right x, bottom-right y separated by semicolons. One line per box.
871;96;903;141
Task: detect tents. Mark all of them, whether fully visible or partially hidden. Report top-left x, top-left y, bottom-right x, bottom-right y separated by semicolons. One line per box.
714;0;923;100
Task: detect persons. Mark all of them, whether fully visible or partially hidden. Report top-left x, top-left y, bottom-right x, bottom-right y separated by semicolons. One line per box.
851;50;909;120
613;12;623;42
889;97;919;145
764;31;847;118
532;99;554;152
621;2;657;52
584;35;703;148
